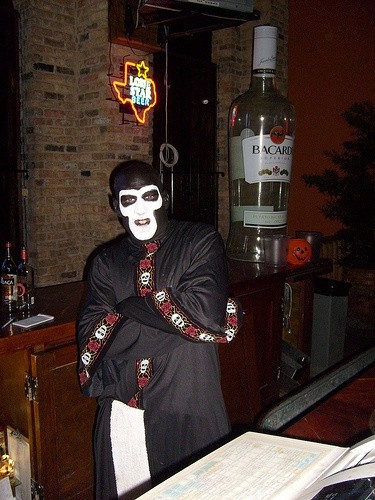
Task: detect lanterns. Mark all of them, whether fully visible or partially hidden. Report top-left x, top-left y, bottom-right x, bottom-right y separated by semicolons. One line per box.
287;239;312;266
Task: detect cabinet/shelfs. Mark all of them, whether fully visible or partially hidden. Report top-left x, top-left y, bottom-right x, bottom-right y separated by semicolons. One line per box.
0;258;333;500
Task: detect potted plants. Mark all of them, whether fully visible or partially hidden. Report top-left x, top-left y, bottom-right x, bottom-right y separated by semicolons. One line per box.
302;101;375;330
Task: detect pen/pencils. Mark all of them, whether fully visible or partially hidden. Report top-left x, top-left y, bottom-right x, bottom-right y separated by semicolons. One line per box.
1;317;16;328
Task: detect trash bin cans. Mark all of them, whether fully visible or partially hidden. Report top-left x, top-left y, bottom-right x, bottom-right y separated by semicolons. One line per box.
311;278;352;369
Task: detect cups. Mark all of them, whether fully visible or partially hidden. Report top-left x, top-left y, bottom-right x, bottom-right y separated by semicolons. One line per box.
296;231;322;261
263;235;289;268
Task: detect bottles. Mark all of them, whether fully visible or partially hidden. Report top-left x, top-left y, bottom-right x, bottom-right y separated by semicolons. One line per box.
18;243;35;320
1;241;19;324
224;24;296;262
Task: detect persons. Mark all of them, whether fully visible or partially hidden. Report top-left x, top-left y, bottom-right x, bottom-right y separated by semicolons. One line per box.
76;160;242;500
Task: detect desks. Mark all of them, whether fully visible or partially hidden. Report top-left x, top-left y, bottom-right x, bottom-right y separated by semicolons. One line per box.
137;432;350;500
